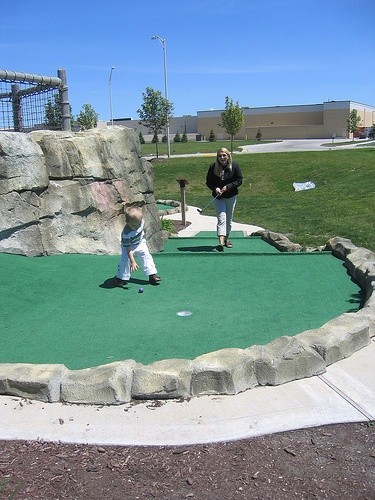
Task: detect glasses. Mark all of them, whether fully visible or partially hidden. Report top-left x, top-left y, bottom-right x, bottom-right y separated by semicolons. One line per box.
219;154;228;157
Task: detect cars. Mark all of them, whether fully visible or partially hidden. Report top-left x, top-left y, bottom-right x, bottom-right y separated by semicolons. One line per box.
365;128;374;137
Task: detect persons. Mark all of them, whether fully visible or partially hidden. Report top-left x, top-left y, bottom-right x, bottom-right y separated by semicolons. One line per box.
206;148;243;251
114;206;161;287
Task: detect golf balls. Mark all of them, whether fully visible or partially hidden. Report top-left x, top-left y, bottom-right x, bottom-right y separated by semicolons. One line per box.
139;288;144;293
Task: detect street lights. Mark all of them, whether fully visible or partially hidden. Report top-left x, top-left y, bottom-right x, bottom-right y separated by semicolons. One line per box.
151;34;170;158
108;66;116;126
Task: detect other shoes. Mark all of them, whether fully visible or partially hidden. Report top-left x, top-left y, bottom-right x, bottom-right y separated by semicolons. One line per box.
216;244;224;253
149;275;161;284
224;240;233;248
116;279;124;287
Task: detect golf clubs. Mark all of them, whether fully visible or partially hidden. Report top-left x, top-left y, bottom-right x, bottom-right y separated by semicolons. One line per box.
197;190;222;213
